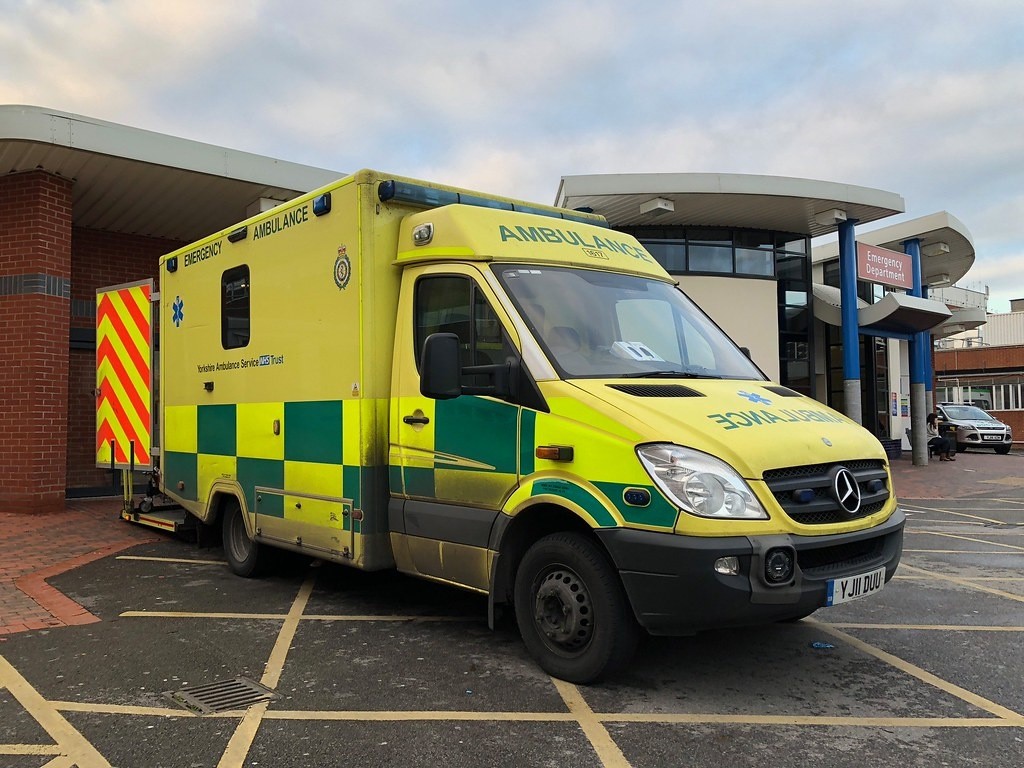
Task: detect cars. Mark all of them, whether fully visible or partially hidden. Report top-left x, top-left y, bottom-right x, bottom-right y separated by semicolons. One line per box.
936;402;1013;456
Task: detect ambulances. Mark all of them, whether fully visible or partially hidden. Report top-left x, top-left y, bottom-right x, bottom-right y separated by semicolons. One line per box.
95;168;906;686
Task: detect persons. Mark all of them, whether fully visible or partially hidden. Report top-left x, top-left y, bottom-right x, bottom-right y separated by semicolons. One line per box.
926;412;957;461
